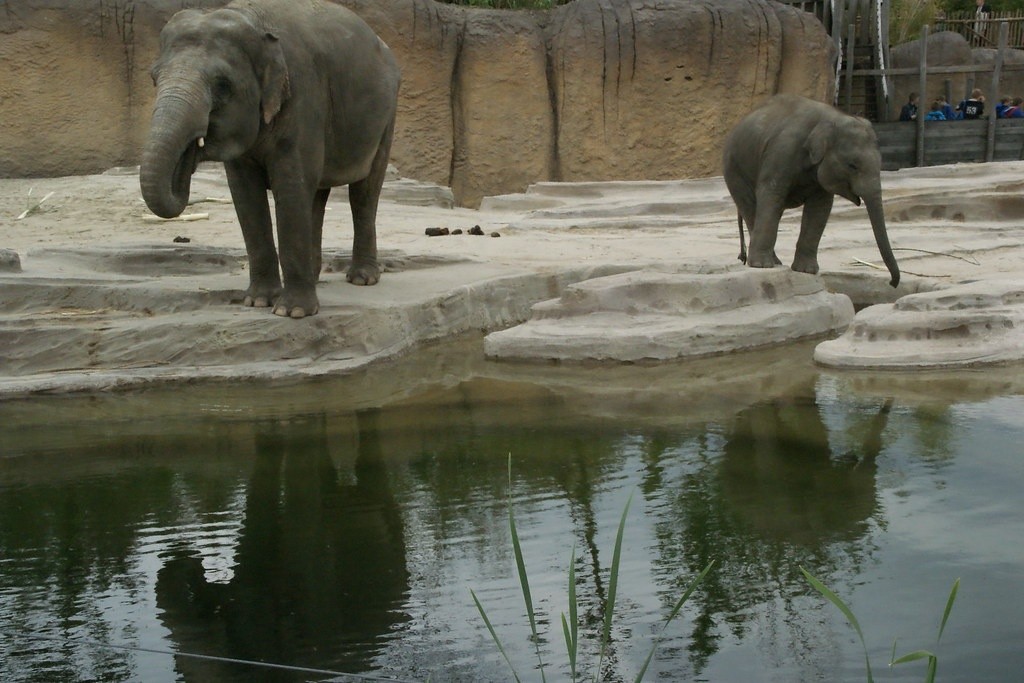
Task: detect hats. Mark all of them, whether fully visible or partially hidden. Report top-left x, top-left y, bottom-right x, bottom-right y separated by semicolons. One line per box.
934;96;944;100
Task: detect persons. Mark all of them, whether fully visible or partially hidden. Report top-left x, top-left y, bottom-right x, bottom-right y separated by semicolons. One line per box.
900;92;919;121
972;0;991;47
927;88;1024;121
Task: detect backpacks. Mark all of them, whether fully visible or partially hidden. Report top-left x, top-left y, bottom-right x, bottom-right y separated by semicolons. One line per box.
986;104;1006;120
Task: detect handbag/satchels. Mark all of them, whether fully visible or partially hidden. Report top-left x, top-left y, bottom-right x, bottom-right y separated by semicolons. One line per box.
956;110;964;120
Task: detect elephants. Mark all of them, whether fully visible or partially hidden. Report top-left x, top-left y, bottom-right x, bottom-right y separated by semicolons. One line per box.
139;1;400;320
719;91;902;291
